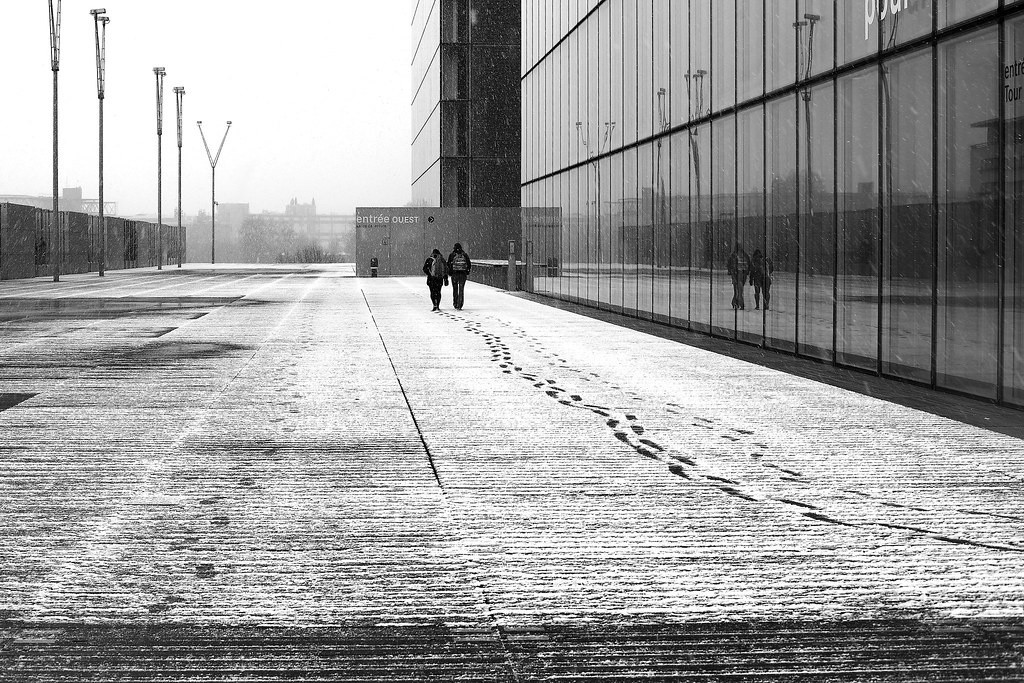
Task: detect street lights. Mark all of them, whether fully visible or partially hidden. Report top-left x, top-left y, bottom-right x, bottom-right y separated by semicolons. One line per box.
195;119;233;264
172;86;187;269
684;69;709;221
656;87;668;191
152;67;167;271
576;120;616;261
89;7;110;285
792;13;821;216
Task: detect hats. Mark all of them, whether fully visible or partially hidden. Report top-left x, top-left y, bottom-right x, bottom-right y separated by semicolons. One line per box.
753;249;762;259
733;242;743;251
433;249;440;254
453;243;462;249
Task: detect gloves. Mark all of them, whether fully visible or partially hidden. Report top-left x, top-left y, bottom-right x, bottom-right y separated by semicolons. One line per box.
444;279;448;286
749;276;754;286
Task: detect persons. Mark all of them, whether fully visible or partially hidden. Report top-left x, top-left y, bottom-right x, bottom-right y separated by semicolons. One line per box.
728;242;773;311
422;249;449;311
448;243;471;309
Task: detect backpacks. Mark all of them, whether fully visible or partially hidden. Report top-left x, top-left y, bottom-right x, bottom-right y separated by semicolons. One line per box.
736;252;750;271
752;259;770;277
452;253;467;271
430;255;447;278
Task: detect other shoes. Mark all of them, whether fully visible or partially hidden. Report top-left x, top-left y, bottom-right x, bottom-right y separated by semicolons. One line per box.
755;307;759;310
432;307;441;311
739;306;744;310
453;303;462;310
